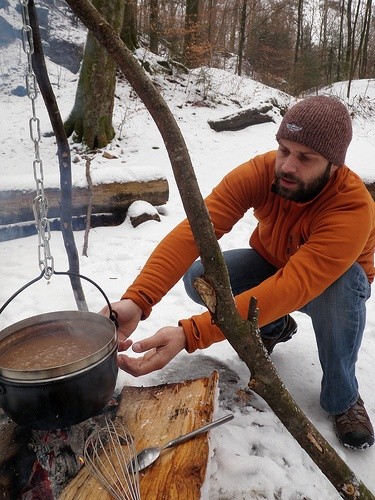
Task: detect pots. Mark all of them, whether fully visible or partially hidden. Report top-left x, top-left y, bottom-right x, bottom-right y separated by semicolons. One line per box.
0;268;119;430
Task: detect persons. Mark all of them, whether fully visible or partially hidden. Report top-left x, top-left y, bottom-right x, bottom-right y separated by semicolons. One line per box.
98;97;375;451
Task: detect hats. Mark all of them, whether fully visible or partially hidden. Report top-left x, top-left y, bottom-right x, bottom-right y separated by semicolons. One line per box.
277;96;352;167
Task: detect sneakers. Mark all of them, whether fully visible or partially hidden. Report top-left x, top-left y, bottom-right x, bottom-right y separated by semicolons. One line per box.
260;313;297;355
332;393;375;450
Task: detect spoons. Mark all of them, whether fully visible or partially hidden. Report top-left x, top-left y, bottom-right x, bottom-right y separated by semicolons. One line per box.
128;413;234;473
